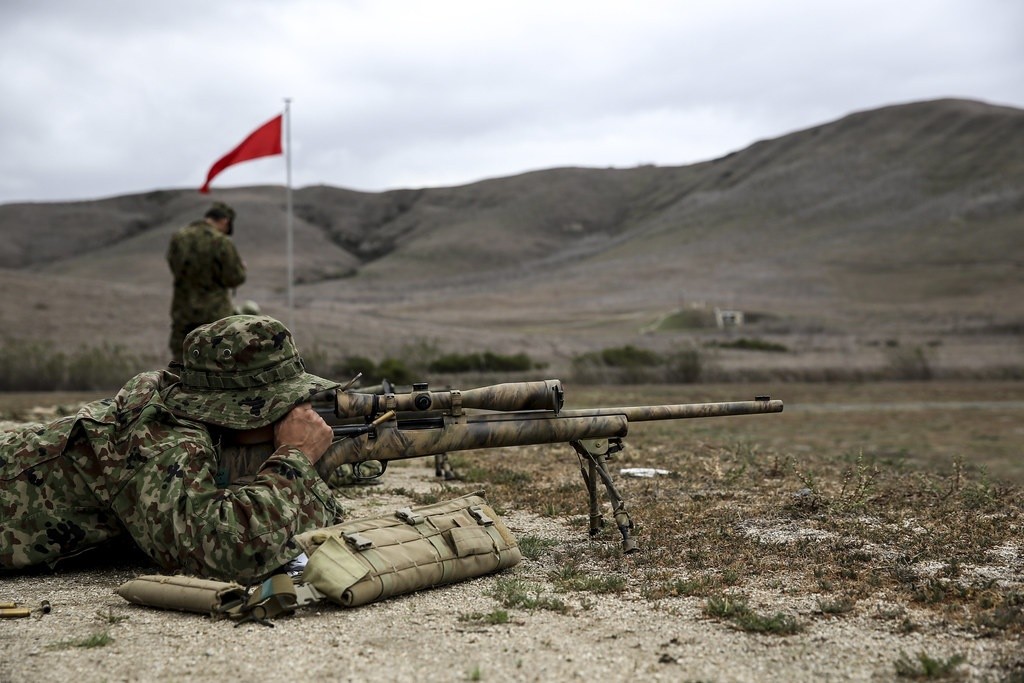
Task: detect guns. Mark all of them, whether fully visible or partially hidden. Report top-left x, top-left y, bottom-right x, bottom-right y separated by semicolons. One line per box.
301;376;784;554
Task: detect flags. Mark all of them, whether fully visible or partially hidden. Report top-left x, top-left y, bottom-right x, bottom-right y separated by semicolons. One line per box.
200;112;283;192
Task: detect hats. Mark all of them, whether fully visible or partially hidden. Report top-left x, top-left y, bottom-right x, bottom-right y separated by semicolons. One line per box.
160;314;340;428
214;200;235;236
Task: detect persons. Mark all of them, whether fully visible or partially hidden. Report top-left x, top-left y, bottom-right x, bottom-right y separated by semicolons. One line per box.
164;200;246;364
0;313;347;585
